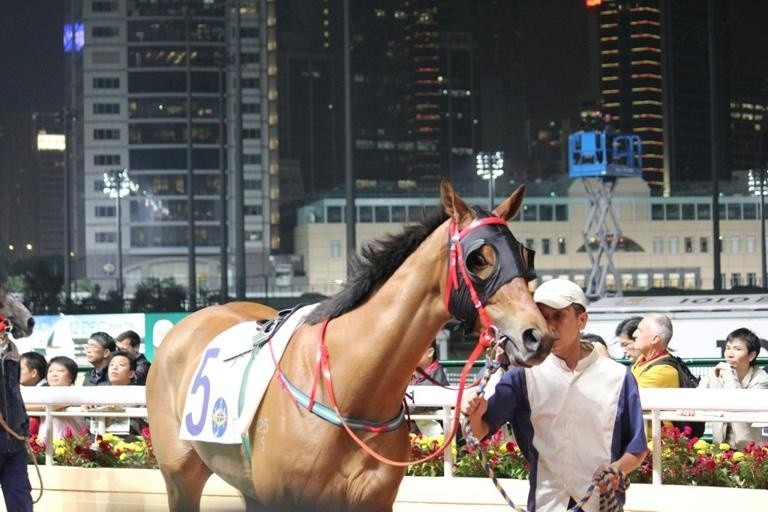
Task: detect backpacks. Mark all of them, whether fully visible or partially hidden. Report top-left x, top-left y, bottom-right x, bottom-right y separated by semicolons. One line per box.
641;356;706;439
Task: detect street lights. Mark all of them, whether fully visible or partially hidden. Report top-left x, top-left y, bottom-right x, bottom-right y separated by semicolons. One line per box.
475;151;504;212
101;169;130;297
745;168;768;296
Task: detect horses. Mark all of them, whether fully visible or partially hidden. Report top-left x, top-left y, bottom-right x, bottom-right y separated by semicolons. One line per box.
143;175;557;512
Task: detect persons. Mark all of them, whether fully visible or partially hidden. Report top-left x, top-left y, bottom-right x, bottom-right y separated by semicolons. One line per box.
18;352;47;455
83;349;144;443
82;331;116;386
459;279;647;512
0;301;34;511
408;337;465;457
578;332;610;355
614;312;679;435
37;355;87;444
115;329;151;374
695;328;768;452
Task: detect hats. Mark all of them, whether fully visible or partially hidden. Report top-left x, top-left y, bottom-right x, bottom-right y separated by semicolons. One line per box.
533;280;588;312
